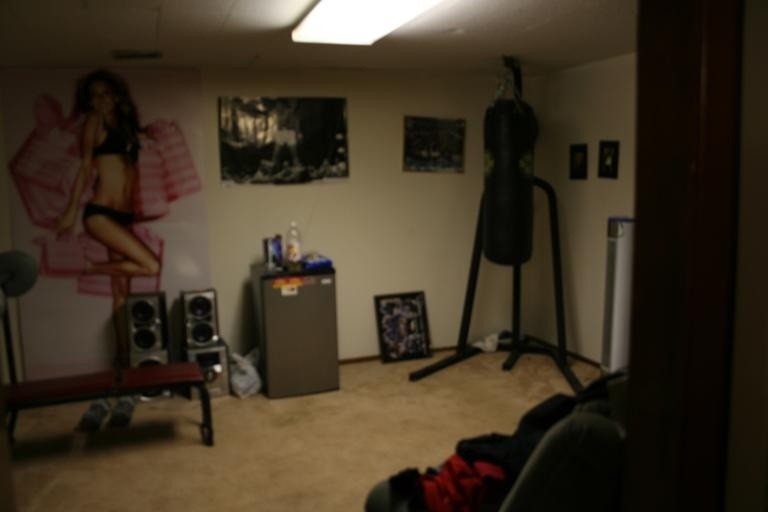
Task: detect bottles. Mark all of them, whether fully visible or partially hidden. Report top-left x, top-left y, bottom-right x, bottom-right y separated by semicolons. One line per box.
285;220;303;273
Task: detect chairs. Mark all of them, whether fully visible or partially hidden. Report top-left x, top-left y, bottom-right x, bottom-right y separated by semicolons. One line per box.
358;410;621;511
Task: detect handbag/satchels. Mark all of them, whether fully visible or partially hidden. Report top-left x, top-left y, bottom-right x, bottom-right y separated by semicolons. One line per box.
230;351;261;400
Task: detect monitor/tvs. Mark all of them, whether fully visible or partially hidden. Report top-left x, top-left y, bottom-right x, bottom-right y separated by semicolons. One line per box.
218;97;348;183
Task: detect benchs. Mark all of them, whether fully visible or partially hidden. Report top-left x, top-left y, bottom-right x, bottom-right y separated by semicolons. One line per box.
1;362;216;449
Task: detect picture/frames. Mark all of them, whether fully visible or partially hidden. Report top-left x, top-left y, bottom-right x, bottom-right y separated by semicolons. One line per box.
372;289;432;366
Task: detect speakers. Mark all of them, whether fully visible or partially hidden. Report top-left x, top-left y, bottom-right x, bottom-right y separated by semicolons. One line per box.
180;288;221;348
127;291;173;402
182;344;231;400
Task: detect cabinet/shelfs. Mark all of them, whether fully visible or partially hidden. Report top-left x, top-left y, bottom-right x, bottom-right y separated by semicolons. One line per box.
249;260;343;402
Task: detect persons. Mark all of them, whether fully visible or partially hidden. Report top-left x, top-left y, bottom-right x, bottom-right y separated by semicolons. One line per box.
57;72;161;368
265;99;302;177
